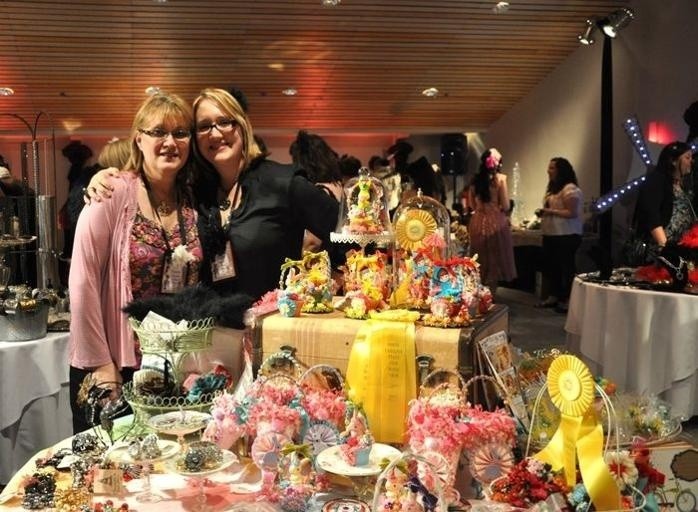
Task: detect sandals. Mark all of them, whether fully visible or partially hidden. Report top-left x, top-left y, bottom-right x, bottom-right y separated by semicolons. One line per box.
533;296;569;315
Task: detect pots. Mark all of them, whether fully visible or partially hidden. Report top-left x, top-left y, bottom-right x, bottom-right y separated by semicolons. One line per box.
0;282;51;343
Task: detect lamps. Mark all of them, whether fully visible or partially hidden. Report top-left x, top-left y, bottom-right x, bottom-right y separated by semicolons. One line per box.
577;7;635;45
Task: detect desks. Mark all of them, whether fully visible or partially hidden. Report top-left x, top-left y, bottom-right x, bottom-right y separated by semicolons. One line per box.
0;226;698;510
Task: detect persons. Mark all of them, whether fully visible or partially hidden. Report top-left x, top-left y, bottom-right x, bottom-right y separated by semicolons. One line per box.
0;139;104;288
341;139;443;202
632;140;698;265
291;132;346;256
469;150;517;301
535;158;583;315
68;88;339;436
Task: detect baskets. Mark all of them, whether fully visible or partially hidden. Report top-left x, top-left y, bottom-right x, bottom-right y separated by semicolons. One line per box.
120;381;229;422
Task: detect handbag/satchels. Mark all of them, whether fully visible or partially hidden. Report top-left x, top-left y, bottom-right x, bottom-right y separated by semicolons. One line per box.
621;239;646;267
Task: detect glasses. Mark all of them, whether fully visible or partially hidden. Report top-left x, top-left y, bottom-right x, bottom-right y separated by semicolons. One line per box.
193;119;238;135
137;128;190;139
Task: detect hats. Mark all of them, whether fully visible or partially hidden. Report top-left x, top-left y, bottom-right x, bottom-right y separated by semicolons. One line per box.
0;166;11;179
663;142;691;160
